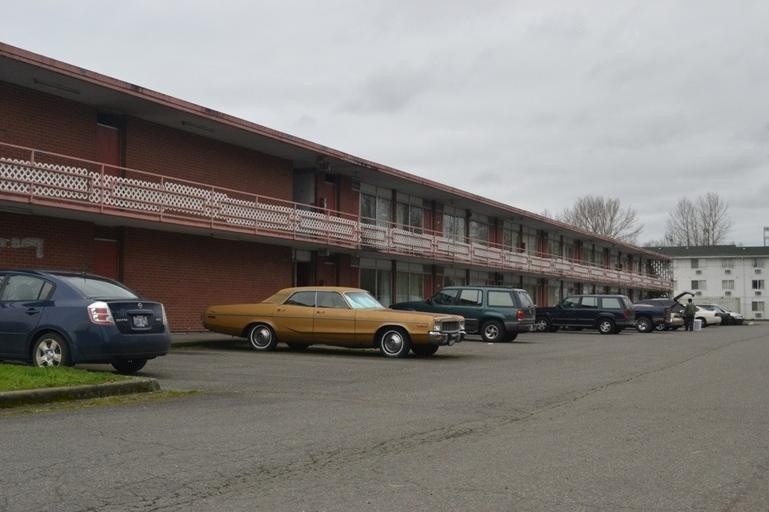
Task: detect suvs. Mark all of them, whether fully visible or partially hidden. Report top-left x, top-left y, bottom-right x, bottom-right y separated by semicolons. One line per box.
389;284;535;342
534;291;695;335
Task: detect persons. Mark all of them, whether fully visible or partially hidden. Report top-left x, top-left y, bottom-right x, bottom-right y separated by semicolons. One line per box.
684;299;696;331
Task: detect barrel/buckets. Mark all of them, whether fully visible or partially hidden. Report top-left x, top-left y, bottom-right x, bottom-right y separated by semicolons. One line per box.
693;319;703;331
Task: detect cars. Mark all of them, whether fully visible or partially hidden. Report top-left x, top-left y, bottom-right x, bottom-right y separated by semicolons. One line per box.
683;302;745;328
201;285;467;359
0;267;172;373
